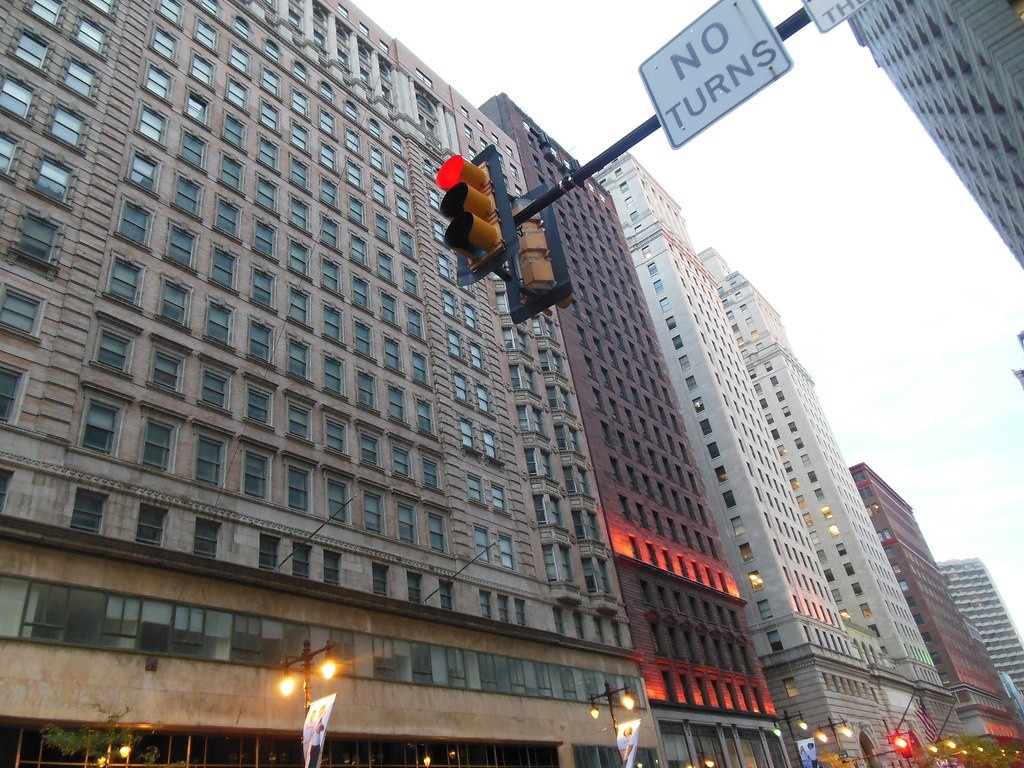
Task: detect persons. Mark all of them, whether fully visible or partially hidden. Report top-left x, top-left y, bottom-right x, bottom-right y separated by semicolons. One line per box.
800;742;817;768
617;726;641;768
304;704;327;768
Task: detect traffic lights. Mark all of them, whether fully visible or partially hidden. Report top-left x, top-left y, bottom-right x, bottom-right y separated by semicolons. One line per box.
436;145;521;286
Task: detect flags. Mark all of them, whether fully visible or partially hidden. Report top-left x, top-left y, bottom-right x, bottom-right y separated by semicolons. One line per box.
303;693;338;768
797;738;818;768
616;719;642;768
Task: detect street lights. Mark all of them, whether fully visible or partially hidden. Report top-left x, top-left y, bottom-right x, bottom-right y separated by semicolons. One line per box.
588;680;634;768
774;711;807;768
281;641;336;768
816;717;847;750
887;728;914;768
928;737;956;768
